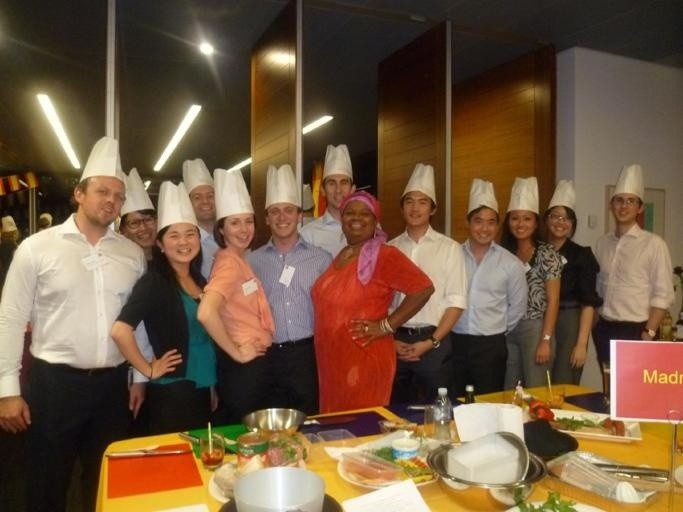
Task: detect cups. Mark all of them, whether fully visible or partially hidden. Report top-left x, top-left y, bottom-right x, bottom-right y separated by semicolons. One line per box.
424;406;439;439
391;439;420;458
545;386;566;409
199;434;225;469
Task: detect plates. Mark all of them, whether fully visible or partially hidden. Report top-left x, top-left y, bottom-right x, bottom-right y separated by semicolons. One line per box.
337;451;435;490
208;455;305;503
216;492;344;512
520;406;642;443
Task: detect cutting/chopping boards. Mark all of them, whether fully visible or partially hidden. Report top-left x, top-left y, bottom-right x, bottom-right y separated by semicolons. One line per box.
187;424;307;463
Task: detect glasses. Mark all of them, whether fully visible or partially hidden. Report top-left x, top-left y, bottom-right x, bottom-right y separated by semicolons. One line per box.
546;212;571;221
126;216;154;229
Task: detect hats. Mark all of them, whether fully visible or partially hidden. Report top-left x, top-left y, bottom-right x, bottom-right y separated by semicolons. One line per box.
181;156;212;190
504;176;540;216
399;161;439;207
264;162;305;210
120;166;155;216
548;178;579;211
211;167;253;219
612;163;646;203
1;211;52;233
322;142;355;181
80;136;126;182
465;176;501;216
157;179;198;230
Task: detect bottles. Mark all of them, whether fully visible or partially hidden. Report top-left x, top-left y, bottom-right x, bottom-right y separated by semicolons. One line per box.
661;310;683;342
435;388;453;445
464;385;476;403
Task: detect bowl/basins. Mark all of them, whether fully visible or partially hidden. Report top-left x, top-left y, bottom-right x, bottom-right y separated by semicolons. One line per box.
233;466;326;512
243;407;305;437
428;431;549;512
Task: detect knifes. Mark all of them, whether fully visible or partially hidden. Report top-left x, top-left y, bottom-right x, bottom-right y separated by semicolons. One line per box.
303;414;358;426
106;448;195;461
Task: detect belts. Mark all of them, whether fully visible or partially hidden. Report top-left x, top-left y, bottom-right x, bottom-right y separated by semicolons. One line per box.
559;304;579;310
271;336;312;351
395;325;438;337
